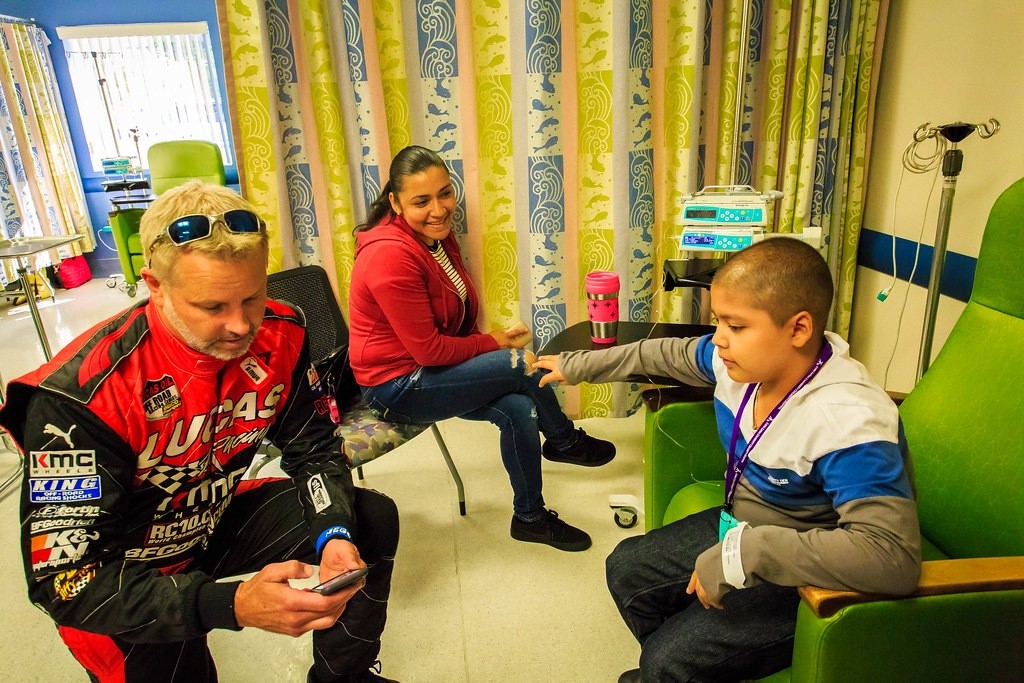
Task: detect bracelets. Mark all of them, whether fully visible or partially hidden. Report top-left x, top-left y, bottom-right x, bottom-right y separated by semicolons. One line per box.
312;525;352;556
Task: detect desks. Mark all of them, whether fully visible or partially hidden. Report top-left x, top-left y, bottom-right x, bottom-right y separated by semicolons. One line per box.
536;321;719;389
0;236;86;364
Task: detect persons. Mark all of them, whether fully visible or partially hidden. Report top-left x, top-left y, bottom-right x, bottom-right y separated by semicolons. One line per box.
532;237;921;683
347;144;616;552
0;177;405;683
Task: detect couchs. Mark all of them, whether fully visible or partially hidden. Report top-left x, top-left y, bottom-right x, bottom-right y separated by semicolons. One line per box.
107;140;226;285
643;177;1023;683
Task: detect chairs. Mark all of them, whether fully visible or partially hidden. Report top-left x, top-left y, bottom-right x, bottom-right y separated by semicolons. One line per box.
253;265;466;516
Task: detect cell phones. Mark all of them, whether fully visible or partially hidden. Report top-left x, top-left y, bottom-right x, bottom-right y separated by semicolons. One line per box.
311;569;368;595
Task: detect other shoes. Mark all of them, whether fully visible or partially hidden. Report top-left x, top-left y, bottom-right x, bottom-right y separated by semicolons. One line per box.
617;668;641;683
306;659;399;683
511;509;591;553
540;428;616;468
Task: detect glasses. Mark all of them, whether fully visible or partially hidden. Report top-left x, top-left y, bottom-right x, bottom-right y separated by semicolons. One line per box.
150;209;265;247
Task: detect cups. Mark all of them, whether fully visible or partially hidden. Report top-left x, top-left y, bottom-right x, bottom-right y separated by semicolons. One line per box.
586;272;620;344
